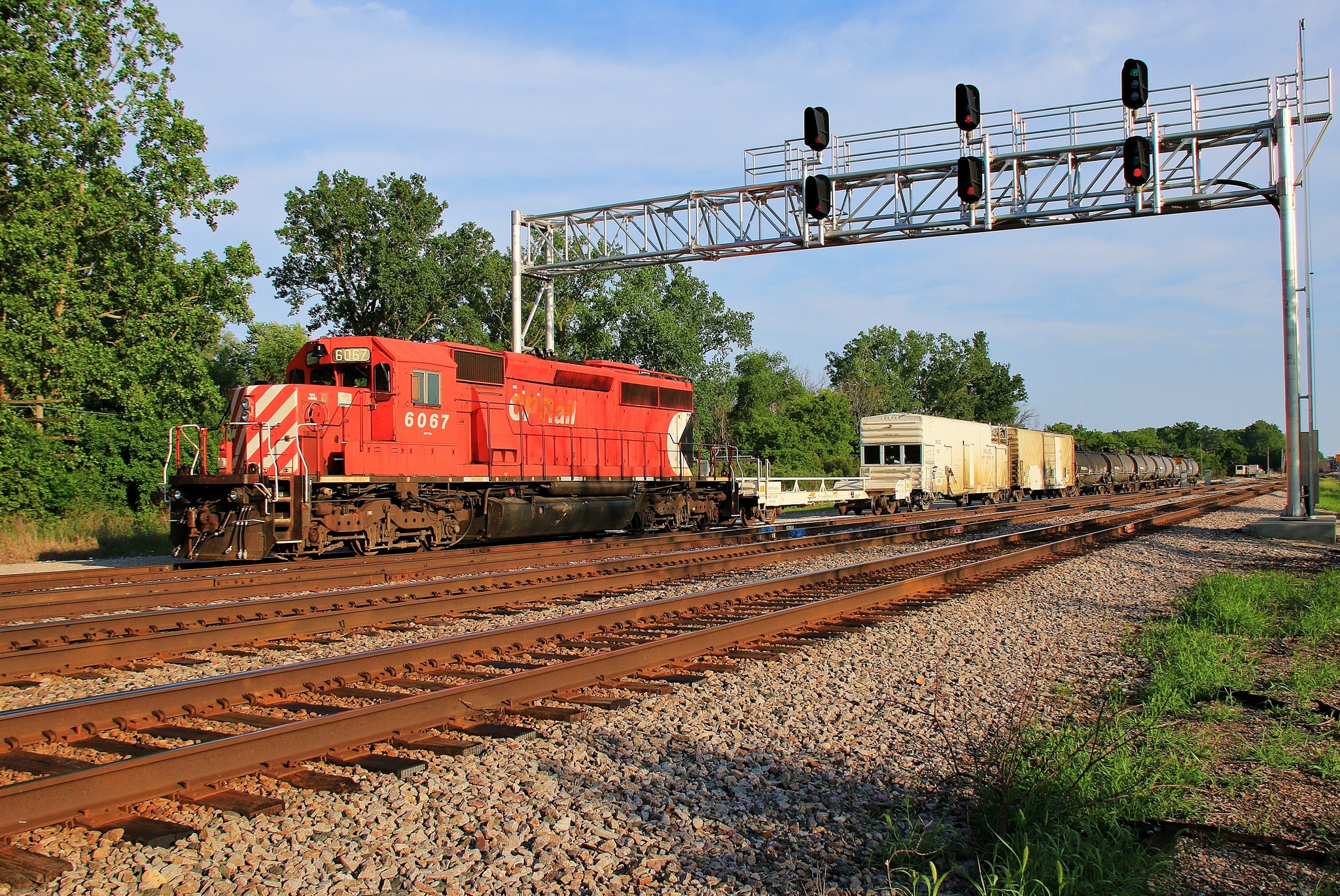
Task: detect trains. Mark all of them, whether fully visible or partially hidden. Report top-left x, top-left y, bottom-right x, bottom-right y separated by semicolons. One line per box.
149;336;1200;566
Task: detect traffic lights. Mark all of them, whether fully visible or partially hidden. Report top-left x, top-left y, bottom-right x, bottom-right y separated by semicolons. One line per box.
804;106;829;152
1123;135;1150;187
1121;58;1148;110
805;174;831;220
957;156;982;204
955;83;981;132
